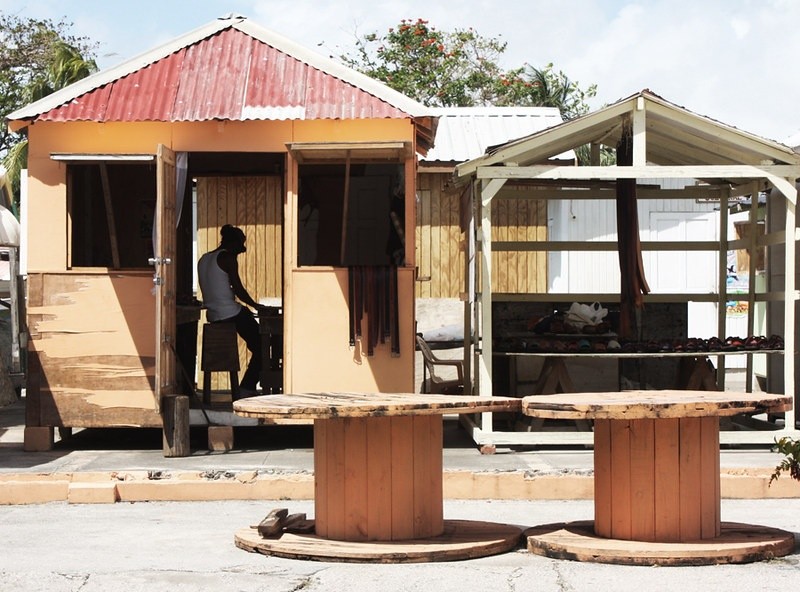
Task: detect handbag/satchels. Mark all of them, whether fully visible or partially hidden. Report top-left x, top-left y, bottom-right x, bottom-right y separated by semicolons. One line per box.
563;301;608;334
533;312;551;334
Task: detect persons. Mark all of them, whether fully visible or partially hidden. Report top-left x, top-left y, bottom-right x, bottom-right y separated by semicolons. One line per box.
197;224;272;399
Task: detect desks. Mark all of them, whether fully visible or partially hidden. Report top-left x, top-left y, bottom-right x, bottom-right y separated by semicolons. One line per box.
232;393;795;567
493;351;799;433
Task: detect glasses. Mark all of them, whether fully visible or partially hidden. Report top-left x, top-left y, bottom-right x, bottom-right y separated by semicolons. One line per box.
238;237;246;253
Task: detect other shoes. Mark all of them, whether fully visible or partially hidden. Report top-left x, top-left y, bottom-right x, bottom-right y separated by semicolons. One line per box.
552;322;577;334
497;334;785;352
583;322;609;335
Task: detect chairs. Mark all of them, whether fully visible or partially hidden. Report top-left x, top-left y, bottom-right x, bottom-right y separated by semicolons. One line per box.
201;324;240;401
415;336;464;394
